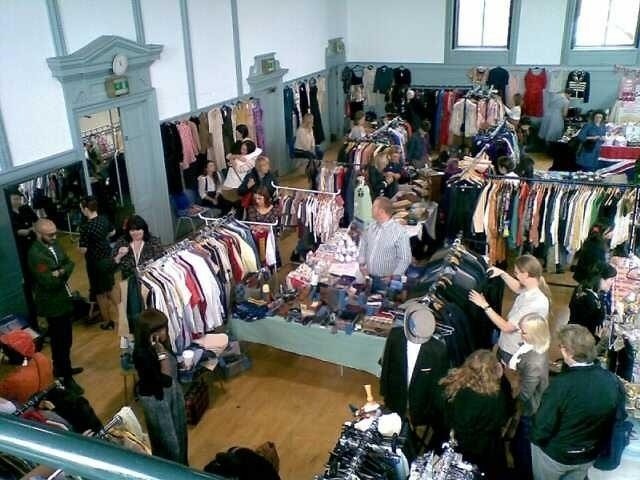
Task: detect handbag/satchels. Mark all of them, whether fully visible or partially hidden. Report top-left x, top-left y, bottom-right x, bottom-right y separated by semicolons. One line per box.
583;138;596;152
185;382;209;424
594;418;632;470
240;191;253;209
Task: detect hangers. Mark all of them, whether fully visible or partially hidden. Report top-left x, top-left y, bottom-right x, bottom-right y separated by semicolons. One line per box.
1;377;125;479
130;214;240;277
423;442;467;479
351;64;406;71
448;151;639;207
338;412;395;479
422;238;470;309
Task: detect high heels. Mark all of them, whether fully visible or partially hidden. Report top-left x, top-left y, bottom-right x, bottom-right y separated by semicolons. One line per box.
101;320;115;330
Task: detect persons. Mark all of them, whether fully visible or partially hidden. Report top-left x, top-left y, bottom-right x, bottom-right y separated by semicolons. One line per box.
576;110;608;171
429;219;628;479
539;88;574;142
356;196;413;298
503;92;521;129
349;110;366;139
406;119;431;167
133;307;189;465
515;157;534;177
404;90;425;127
204;442;280;480
516;117;531;159
289;113;324;160
494;155;519;188
196;124;281;275
0;188;165;402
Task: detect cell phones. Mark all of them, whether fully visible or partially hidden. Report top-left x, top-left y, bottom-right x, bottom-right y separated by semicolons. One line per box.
153;336;161;342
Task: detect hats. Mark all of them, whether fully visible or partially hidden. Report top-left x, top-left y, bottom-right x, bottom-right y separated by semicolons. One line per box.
592;109;607;120
0;330;35;359
403;303;438;344
445;160;462;175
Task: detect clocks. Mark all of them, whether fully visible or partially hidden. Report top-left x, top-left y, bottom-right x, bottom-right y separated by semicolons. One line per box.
109;53;132;75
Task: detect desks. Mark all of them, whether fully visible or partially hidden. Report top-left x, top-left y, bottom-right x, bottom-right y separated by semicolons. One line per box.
229;262;401;376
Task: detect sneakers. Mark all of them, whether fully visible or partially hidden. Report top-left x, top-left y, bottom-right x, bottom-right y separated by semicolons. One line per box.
69;381;83;395
70;367;83;375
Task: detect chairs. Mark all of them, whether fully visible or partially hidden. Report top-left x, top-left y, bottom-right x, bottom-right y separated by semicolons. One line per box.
172;191;209;235
285;136;324;173
